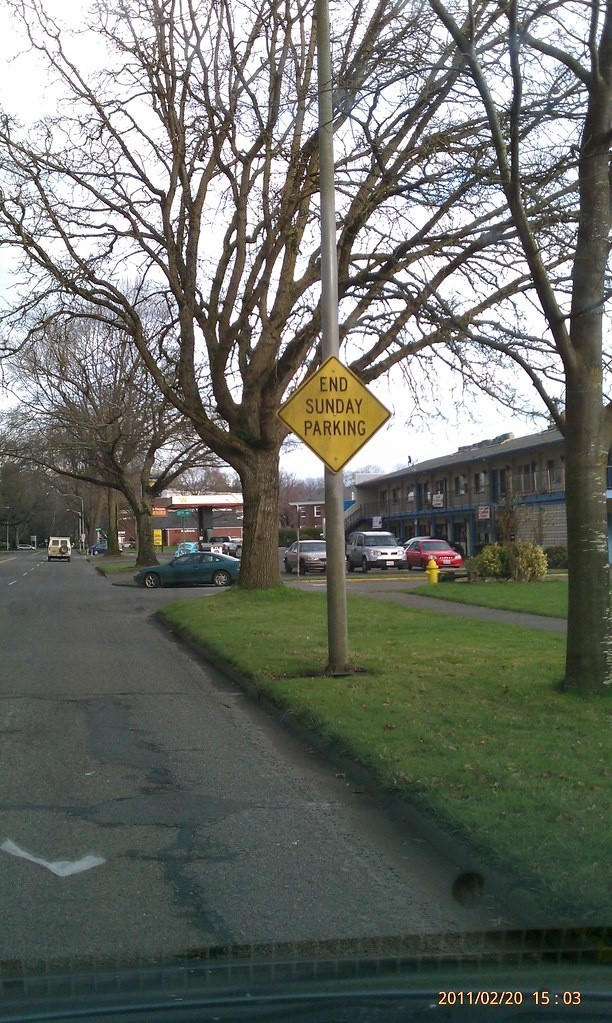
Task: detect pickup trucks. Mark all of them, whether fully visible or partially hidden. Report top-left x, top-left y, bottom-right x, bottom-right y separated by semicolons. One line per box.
230;536;243;547
200;535;238;558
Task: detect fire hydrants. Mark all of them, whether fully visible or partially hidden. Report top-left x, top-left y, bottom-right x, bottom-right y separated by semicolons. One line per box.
424;559;441;585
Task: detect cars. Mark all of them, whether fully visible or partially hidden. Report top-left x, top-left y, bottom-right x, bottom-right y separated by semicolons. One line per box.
133;552;241;588
404;536;464;571
124;541;136;548
282;539;326;575
346;531;407;573
174;542;200;557
88;543;123;555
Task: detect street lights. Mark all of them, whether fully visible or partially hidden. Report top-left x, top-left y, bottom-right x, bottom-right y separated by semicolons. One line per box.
290;504;300;578
63;493;85;552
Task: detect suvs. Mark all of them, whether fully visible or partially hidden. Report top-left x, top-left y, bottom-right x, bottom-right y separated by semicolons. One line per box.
47;536;74;562
17;544;35;551
